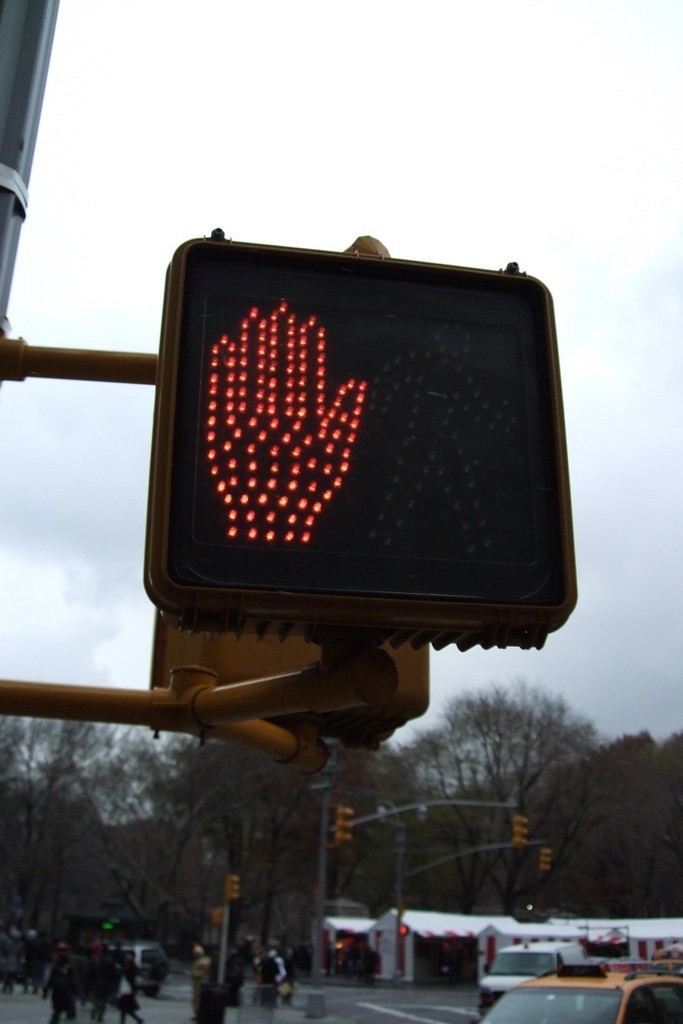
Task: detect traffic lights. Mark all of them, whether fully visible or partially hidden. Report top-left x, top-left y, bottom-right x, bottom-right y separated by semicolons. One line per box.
512;813;530;850
399;925;409;938
334;802;356;844
145;228;578;651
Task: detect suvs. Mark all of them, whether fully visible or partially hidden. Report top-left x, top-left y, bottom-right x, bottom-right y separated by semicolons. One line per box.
478;938;590;1010
104;939;170;997
476;958;683;1023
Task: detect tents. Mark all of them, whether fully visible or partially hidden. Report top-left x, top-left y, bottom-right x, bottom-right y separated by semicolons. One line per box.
320;916;380;974
367;908;683;989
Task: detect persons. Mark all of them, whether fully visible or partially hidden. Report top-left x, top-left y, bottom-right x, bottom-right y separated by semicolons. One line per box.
285;941;376;985
669;944;682;959
185;934;294;1024
445;949;464;987
42;940;147;1024
0;928;46;994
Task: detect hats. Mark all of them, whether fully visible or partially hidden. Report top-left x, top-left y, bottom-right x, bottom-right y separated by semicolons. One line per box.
267;950;278;958
54;942;68;958
26;929;37;940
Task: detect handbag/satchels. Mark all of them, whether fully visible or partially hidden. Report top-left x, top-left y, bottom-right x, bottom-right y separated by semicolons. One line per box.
277;982;292;996
116;976;131;997
